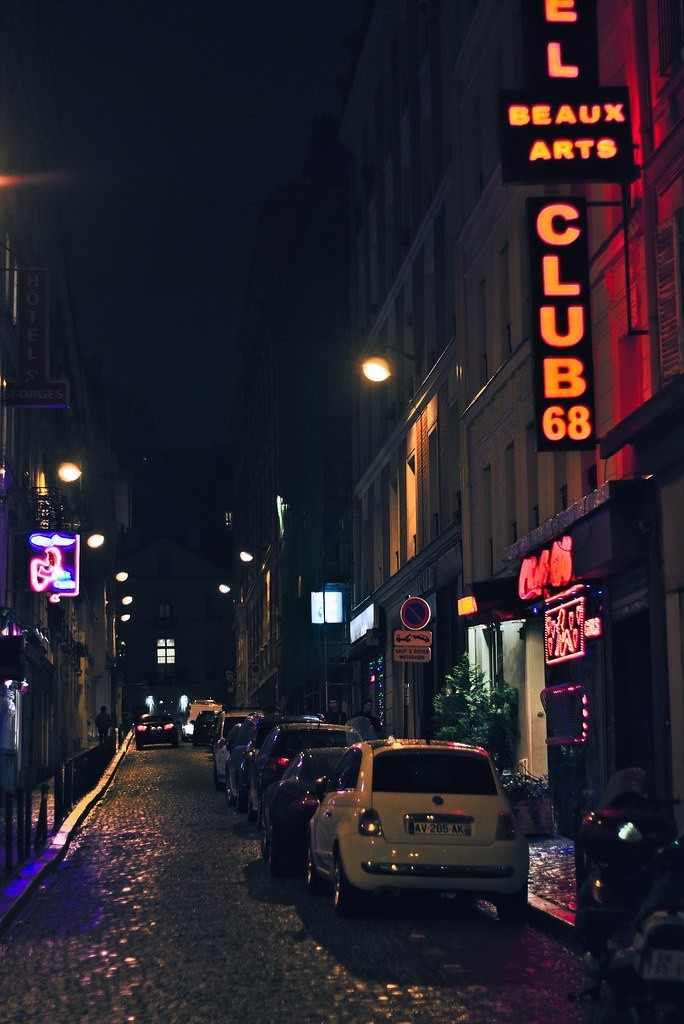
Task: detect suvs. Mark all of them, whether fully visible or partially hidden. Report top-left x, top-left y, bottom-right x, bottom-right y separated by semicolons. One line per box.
190;710;224;746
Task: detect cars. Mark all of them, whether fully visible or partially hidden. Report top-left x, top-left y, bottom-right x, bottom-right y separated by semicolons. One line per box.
132;714;178;750
304;740;530;923
211;711;365;876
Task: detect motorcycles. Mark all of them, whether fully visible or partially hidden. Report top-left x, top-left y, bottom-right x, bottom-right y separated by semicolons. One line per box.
567;767;684;1024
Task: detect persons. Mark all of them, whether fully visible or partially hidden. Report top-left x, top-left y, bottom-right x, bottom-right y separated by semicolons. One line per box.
352;699;379;732
95;706;110;744
327;697;347;726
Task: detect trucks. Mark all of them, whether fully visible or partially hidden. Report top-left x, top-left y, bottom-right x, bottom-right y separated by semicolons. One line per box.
181;700;222;743
